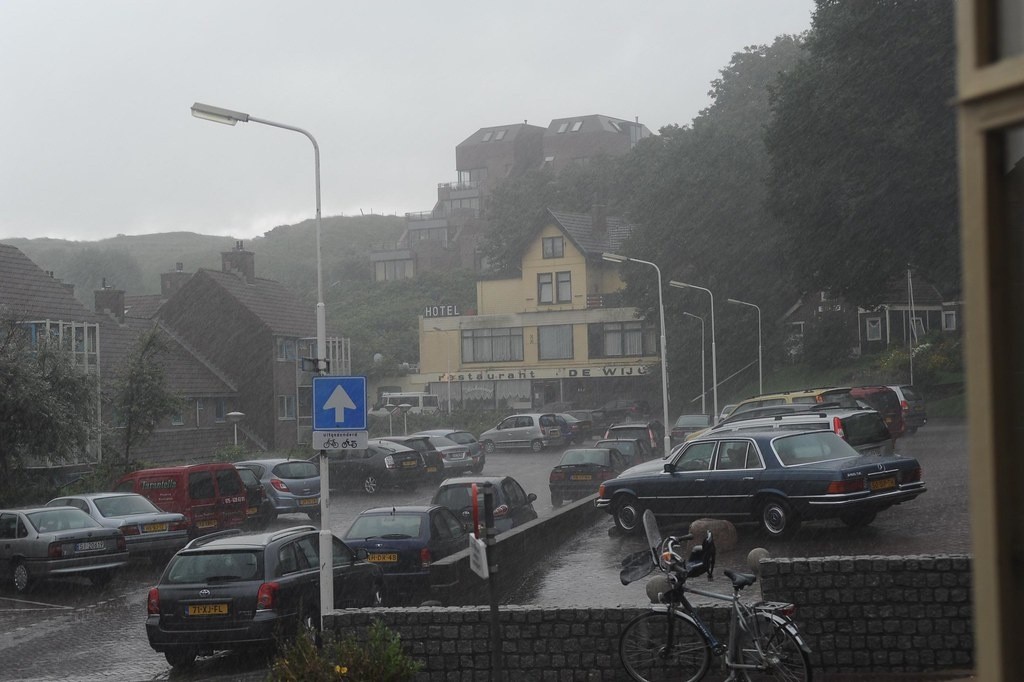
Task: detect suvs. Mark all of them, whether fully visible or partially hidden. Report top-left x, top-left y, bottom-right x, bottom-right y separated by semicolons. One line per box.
670;384;928;453
146;525;392;668
603;420;665;458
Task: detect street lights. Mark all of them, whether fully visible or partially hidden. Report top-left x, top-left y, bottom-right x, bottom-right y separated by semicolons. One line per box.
191;102;333;631
601;253;670;458
669;280;763;426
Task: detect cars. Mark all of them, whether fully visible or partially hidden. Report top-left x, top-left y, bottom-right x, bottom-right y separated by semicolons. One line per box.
0;506;129;593
307;429;485;495
549;435;657;506
44;492;189;572
342;504;486;598
596;429;927;541
431;476;538;537
481;398;652;454
229;442;321;531
670;415;714;449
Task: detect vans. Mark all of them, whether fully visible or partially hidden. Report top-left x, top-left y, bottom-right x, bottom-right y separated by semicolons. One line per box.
113;462;248;541
368;392;440;418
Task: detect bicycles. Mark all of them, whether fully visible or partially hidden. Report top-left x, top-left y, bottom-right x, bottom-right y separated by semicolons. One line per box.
619;534;813;682
